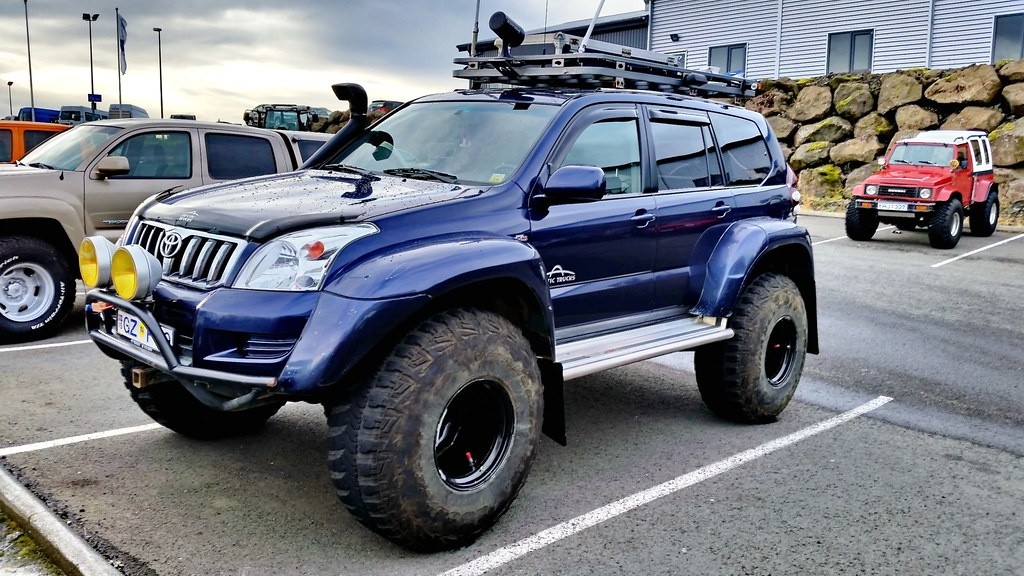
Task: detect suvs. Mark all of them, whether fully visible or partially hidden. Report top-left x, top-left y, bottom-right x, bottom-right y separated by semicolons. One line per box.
845;130;1000;250
79;0;820;555
0;116;337;344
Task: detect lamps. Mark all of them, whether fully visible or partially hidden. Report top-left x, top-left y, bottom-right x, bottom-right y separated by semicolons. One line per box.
670;33;679;41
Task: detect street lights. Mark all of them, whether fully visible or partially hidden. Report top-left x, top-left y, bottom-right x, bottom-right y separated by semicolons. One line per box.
7;82;13;115
82;13;100;121
153;28;164;118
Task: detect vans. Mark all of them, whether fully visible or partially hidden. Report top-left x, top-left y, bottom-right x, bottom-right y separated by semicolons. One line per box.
108;104;150;119
59;106;109;127
